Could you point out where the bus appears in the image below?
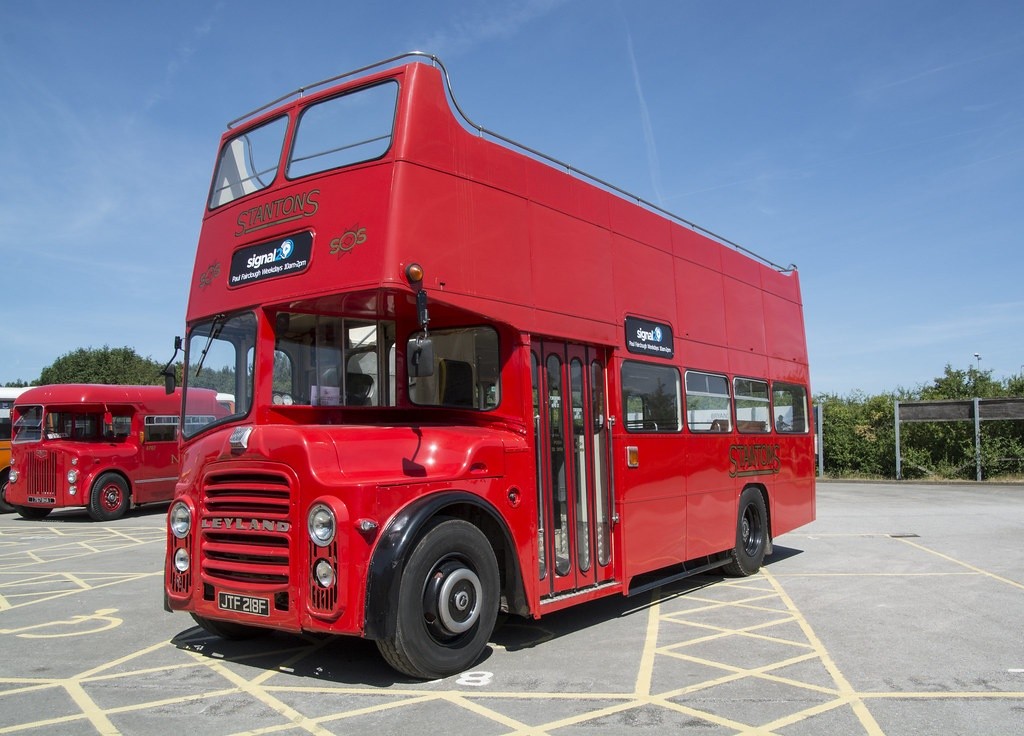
[163,47,822,682]
[5,384,232,523]
[0,386,241,515]
